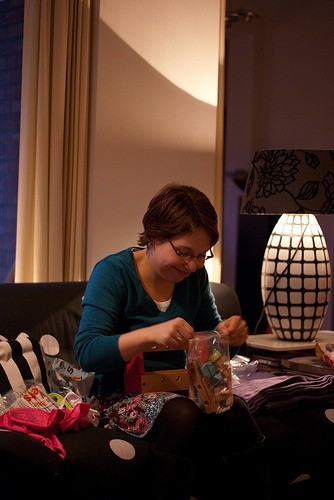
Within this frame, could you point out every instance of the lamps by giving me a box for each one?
[239,148,332,342]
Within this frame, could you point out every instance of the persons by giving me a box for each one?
[73,183,261,500]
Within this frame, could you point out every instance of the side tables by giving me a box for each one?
[245,330,334,375]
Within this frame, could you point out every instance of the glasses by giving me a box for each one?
[168,239,213,260]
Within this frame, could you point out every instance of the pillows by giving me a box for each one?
[0,337,52,408]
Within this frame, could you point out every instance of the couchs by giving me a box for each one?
[0,282,334,475]
